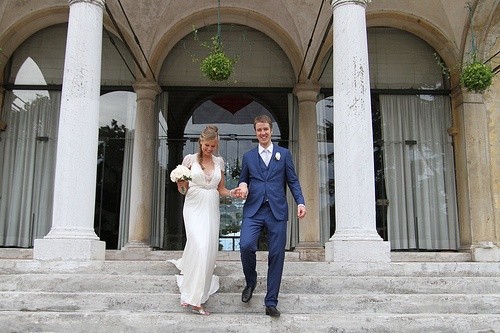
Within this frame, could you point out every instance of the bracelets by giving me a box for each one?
[230,190,233,197]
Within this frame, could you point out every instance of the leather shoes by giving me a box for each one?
[266,305,280,316]
[242,285,256,302]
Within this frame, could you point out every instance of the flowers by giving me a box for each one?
[275,152,281,161]
[170,165,193,193]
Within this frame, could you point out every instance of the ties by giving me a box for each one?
[264,149,270,165]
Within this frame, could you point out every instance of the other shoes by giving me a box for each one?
[180,303,189,307]
[192,306,207,314]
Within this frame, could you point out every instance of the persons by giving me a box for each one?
[236,115,307,317]
[168,126,248,315]
